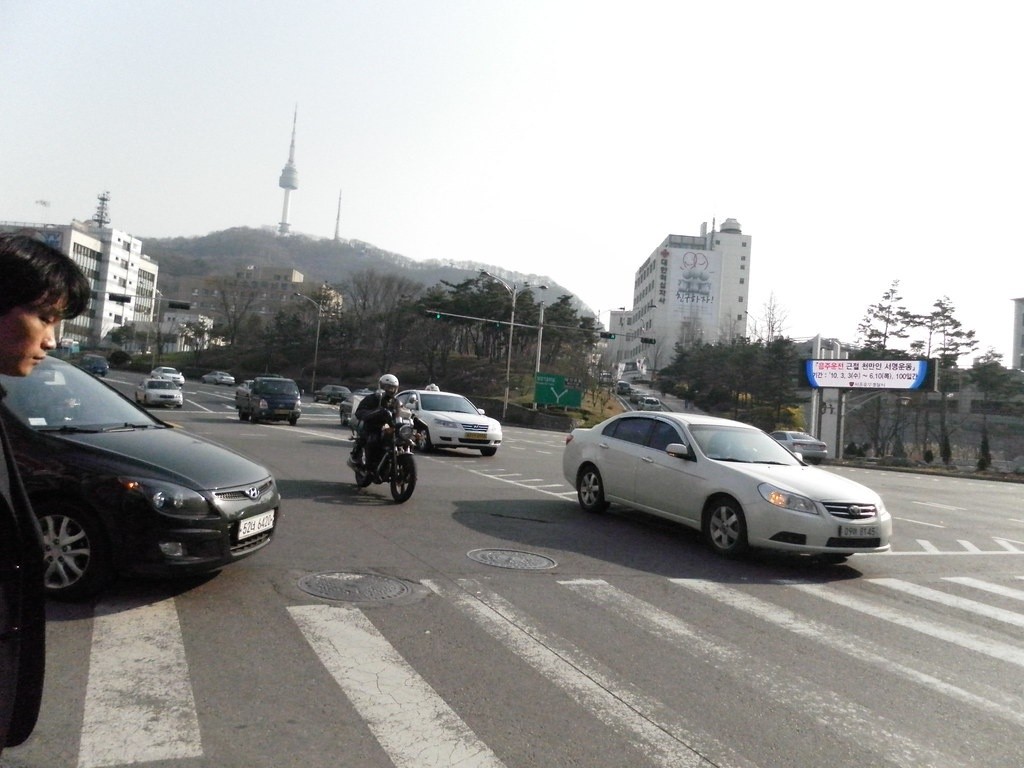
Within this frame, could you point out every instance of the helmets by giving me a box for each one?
[379,374,400,398]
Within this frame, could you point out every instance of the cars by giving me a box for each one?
[73,355,110,376]
[135,366,186,407]
[0,354,281,600]
[393,385,503,457]
[637,397,661,411]
[768,431,829,464]
[236,380,276,399]
[562,410,893,565]
[201,370,234,386]
[599,369,649,402]
[313,385,383,426]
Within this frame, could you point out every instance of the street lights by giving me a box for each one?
[745,311,757,342]
[587,305,657,400]
[294,283,336,394]
[891,395,912,457]
[481,271,548,420]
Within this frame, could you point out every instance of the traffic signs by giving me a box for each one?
[532,371,583,408]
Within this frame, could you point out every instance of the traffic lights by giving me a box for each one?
[641,337,656,344]
[600,332,615,339]
[426,312,441,319]
[485,322,500,328]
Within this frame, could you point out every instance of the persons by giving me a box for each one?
[1,234,88,748]
[355,374,408,475]
[426,377,440,392]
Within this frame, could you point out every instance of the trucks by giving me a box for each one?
[233,377,302,426]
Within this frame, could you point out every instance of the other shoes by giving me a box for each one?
[350,446,363,464]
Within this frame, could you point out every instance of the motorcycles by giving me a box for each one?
[347,395,418,502]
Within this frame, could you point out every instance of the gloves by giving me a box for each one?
[375,406,393,423]
